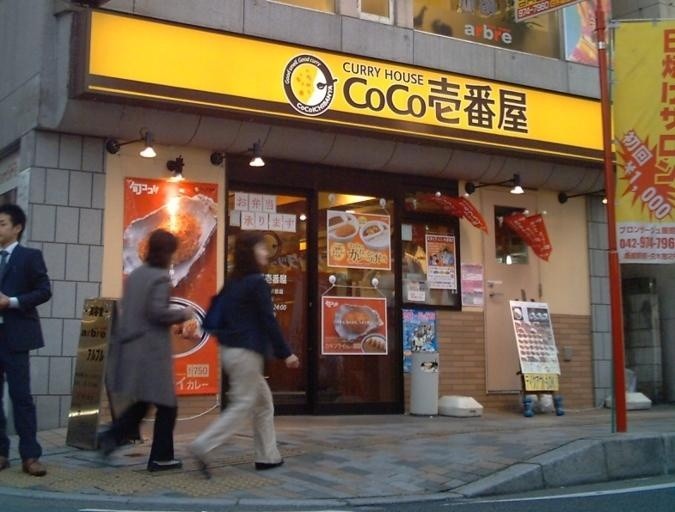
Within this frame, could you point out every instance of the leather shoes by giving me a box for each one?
[148,459,181,471]
[255,459,283,470]
[23,458,46,476]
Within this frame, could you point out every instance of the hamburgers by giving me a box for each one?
[341,311,371,333]
[138,214,200,266]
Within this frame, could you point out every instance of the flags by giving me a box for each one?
[612,16,675,265]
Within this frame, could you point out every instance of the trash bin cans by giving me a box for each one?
[409,352,440,416]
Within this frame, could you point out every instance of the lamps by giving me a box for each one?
[167,156,186,183]
[462,172,522,199]
[555,187,609,209]
[206,138,265,171]
[103,126,158,160]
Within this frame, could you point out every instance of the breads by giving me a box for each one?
[364,337,386,353]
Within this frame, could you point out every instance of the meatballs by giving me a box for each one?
[172,325,182,333]
[183,320,197,337]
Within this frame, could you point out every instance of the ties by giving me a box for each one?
[0,251,8,273]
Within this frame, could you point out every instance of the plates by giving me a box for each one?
[329,213,390,250]
[166,296,210,358]
[359,334,386,353]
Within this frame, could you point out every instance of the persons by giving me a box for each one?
[95,225,196,474]
[188,229,302,482]
[1,204,56,479]
